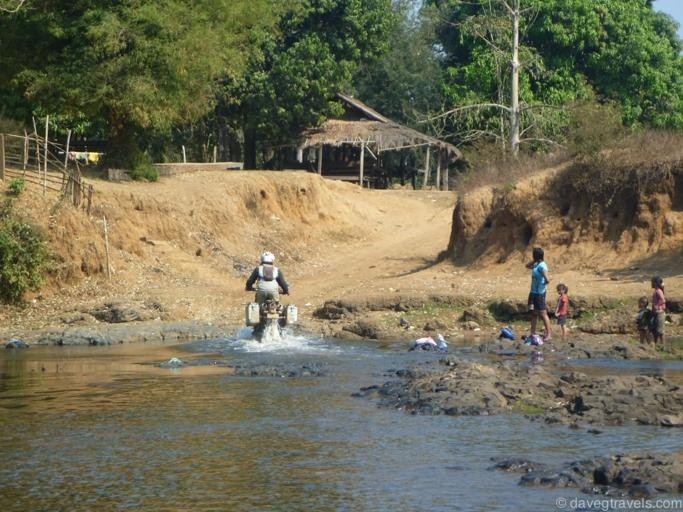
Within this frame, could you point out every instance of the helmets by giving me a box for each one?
[261,251,275,263]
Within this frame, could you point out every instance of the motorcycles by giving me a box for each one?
[245,288,298,343]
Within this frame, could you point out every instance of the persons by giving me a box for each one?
[651,276,666,345]
[245,252,289,336]
[638,296,650,345]
[525,248,552,341]
[555,284,568,340]
